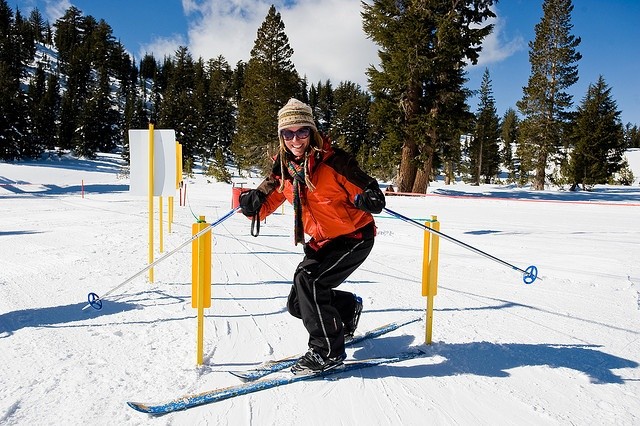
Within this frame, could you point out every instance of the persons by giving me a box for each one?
[239,96,386,378]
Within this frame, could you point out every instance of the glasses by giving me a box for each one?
[280,126,312,141]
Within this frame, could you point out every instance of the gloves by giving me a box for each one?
[240,190,261,217]
[362,185,385,214]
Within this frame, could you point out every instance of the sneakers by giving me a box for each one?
[290,348,346,376]
[342,294,362,341]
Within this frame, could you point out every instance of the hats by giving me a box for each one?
[277,98,316,193]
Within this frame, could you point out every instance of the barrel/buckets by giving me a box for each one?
[232,187,251,213]
